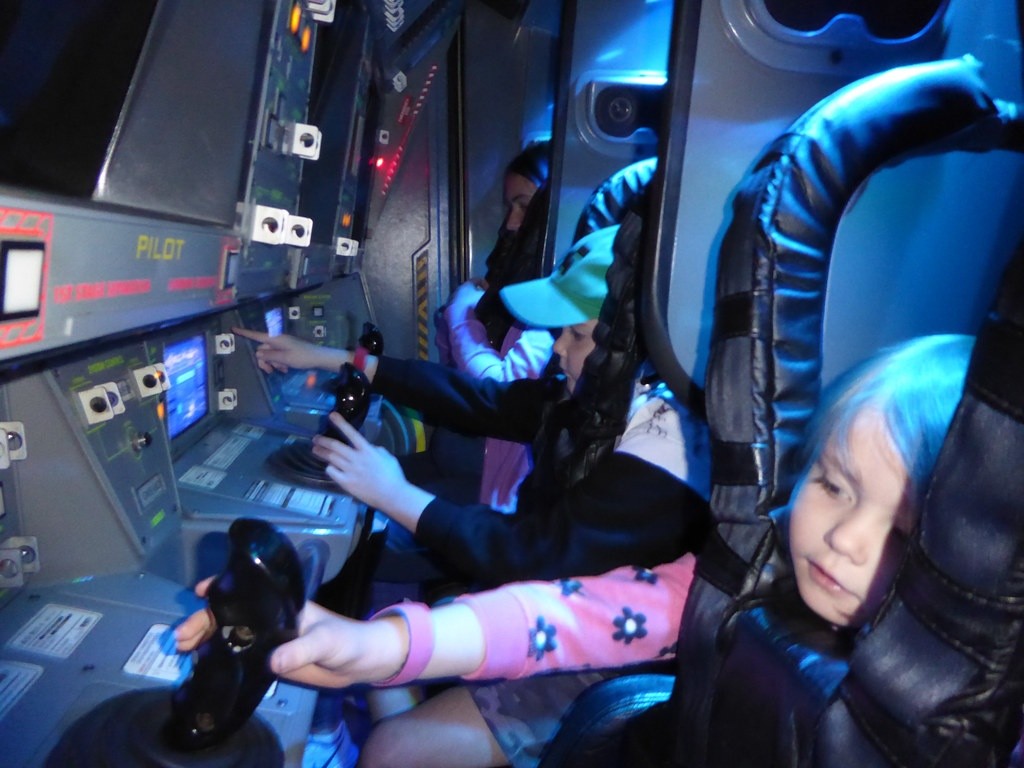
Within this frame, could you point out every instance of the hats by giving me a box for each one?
[497,223,621,330]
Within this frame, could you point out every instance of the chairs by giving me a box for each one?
[527,59,1024,766]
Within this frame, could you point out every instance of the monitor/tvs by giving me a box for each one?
[161,325,215,451]
[259,301,287,339]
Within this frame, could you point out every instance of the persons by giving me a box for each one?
[217,225,716,768]
[168,335,978,768]
[349,231,558,587]
[476,138,552,290]
